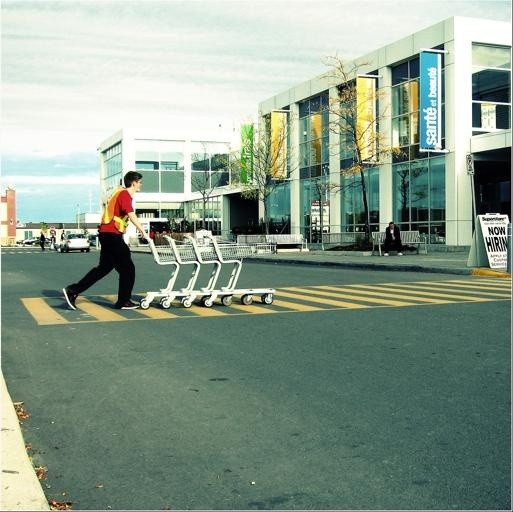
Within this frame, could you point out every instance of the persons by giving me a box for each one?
[61,171,148,311]
[39,232,45,250]
[384,222,402,256]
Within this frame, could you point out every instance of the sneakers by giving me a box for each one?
[382,252,404,257]
[63,284,80,311]
[113,299,140,310]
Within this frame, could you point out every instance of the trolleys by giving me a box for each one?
[140,232,277,310]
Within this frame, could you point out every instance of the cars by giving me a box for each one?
[92,240,96,245]
[60,233,90,252]
[430,230,445,243]
[17,237,51,245]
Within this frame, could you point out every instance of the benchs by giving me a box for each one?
[237,234,309,253]
[322,231,427,257]
